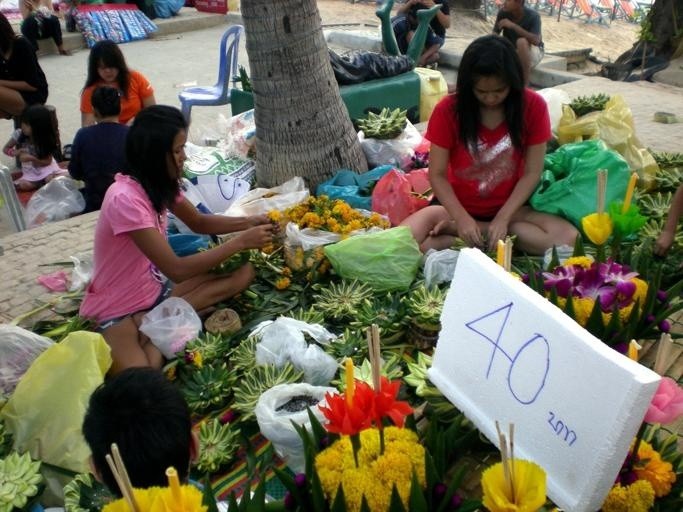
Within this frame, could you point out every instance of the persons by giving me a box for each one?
[80,106,280,374]
[18,0,69,55]
[391,0,450,69]
[0,13,48,119]
[399,34,582,259]
[652,182,683,258]
[83,365,202,501]
[2,105,60,191]
[493,0,545,80]
[79,40,156,127]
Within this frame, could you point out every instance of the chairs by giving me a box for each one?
[178,26,243,139]
